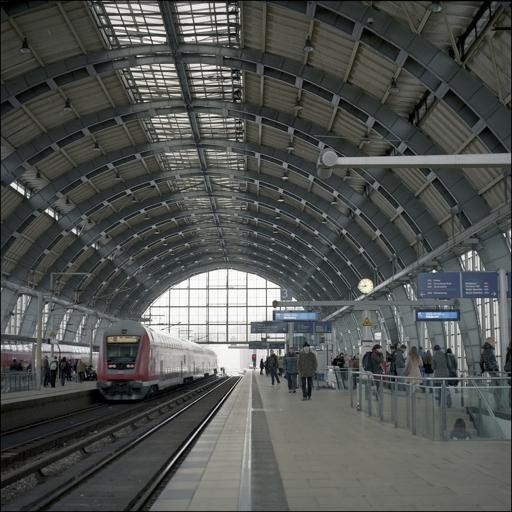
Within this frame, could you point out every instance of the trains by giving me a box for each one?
[95,319,220,401]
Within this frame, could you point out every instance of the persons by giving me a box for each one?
[478,336,505,410]
[10,355,97,388]
[331,341,458,408]
[449,417,471,441]
[259,342,323,400]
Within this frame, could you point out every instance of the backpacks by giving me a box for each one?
[362,352,372,371]
[60,362,66,370]
[268,355,276,370]
[50,362,57,370]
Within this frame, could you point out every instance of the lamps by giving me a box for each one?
[391,76,400,93]
[92,139,99,151]
[287,141,294,150]
[19,37,31,54]
[357,277,375,296]
[344,168,352,179]
[302,35,316,53]
[104,233,110,240]
[115,172,120,180]
[87,218,93,225]
[361,129,372,145]
[293,96,304,110]
[143,212,150,220]
[331,197,338,206]
[131,195,136,203]
[281,170,289,181]
[63,96,73,113]
[429,1,442,14]
[35,171,42,180]
[65,195,72,206]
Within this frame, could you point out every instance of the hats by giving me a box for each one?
[485,337,496,349]
[303,342,310,347]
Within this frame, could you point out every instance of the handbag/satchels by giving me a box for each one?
[403,367,409,376]
[391,360,395,370]
[423,364,433,373]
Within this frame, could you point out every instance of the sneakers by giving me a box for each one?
[302,396,312,400]
[288,390,296,393]
